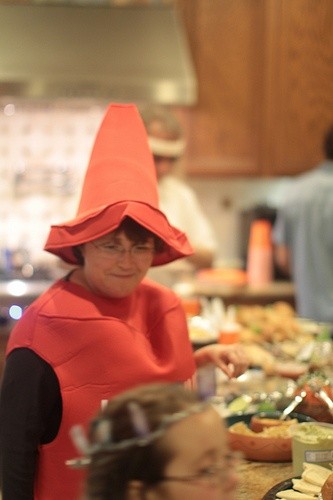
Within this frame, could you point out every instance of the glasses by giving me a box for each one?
[91,240,159,261]
[162,451,239,488]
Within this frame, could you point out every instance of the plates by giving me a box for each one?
[261,476,305,500]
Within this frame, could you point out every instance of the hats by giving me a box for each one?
[43,102,194,266]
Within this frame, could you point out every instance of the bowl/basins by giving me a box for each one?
[223,411,318,462]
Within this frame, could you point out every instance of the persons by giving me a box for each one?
[271,121,333,324]
[144,110,218,269]
[88,383,239,500]
[0,193,197,500]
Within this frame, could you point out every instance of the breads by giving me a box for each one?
[276,463,333,500]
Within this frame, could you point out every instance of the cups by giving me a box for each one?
[291,421,333,475]
